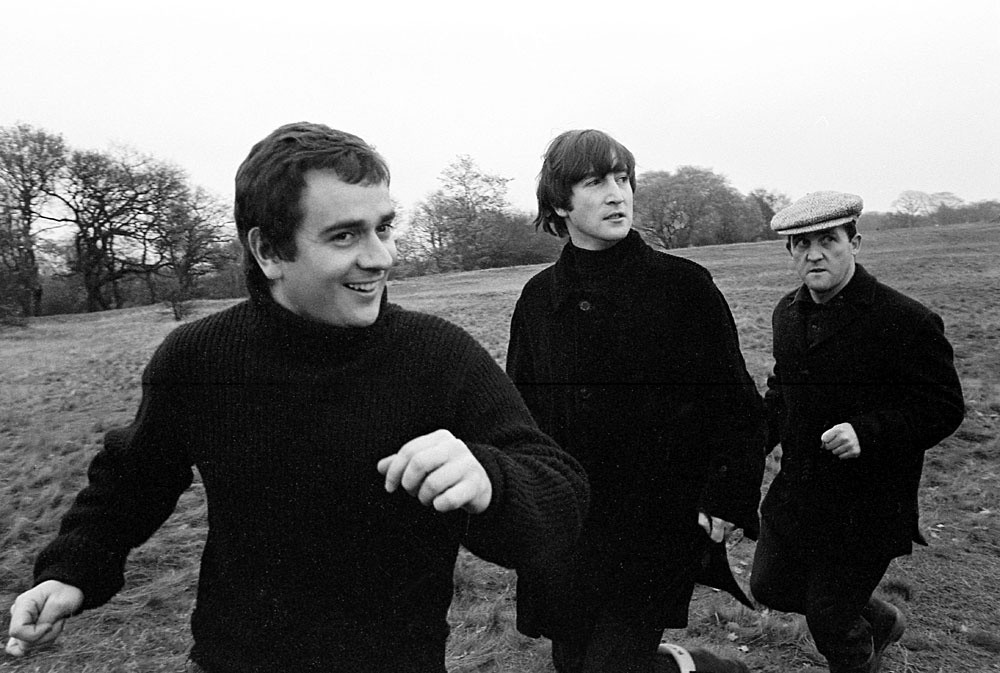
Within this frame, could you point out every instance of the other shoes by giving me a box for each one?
[870,600,907,668]
[721,657,749,673]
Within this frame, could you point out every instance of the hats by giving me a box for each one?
[771,191,864,238]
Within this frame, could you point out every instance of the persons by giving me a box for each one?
[505,131,767,673]
[7,120,592,673]
[750,190,962,673]
